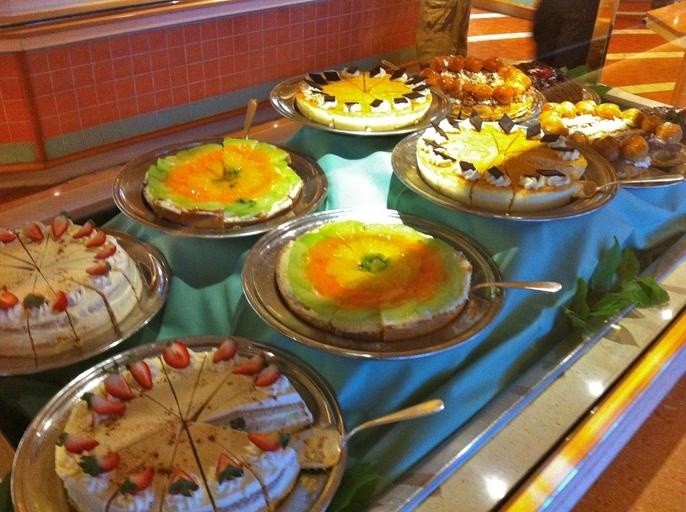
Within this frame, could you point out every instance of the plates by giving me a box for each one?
[389,117,618,224]
[269,66,439,134]
[420,49,543,126]
[2,212,176,377]
[112,133,331,241]
[244,203,509,364]
[8,336,348,511]
[620,106,686,194]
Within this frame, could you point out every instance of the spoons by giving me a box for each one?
[473,280,567,295]
[589,172,686,199]
[285,393,453,481]
[238,100,267,139]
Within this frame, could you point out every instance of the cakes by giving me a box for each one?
[54,337,312,512]
[539,98,686,175]
[275,219,472,341]
[142,136,303,230]
[415,108,587,214]
[295,63,433,133]
[516,59,584,104]
[0,214,142,357]
[419,54,537,123]
